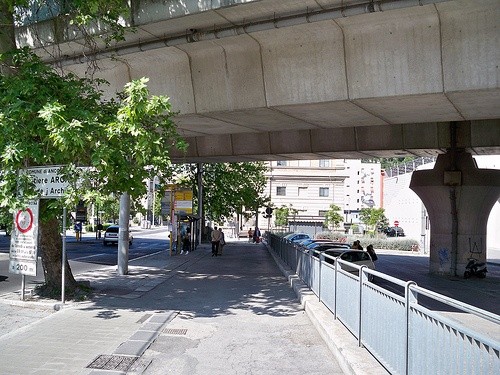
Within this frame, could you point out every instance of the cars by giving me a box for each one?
[282,233,350,261]
[103,226,133,246]
[315,248,376,282]
[388,226,403,236]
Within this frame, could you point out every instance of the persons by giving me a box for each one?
[247,227,253,242]
[97,223,103,239]
[210,227,222,257]
[351,241,358,260]
[252,227,261,244]
[364,245,380,284]
[356,239,364,260]
[74,219,83,242]
[217,228,224,256]
[180,227,192,256]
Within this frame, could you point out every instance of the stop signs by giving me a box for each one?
[394,221,399,226]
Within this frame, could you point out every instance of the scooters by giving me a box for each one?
[463,257,488,279]
